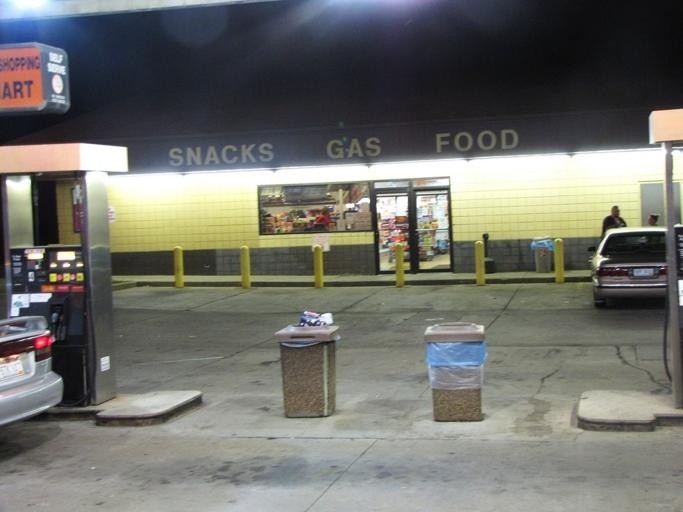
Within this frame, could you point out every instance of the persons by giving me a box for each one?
[599,206,627,242]
[648,214,659,227]
[315,207,330,226]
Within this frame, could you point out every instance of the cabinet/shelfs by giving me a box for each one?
[381,228,439,262]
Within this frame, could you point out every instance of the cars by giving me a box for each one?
[0,314,64,427]
[588,225,668,308]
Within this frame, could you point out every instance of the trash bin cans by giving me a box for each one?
[275,324,342,418]
[531,239,552,273]
[424,320,485,422]
